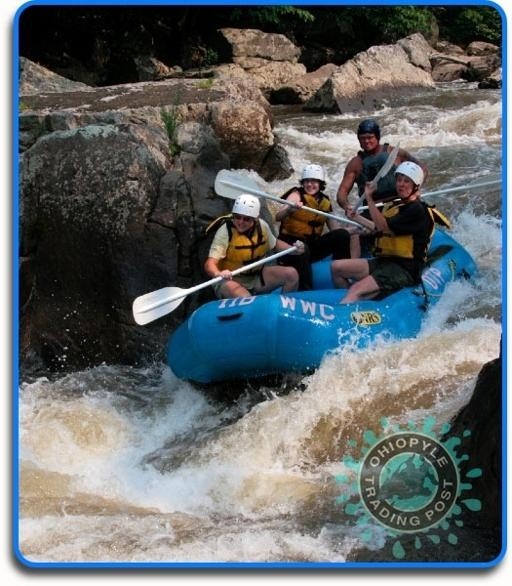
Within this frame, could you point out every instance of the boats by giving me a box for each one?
[168,224,478,390]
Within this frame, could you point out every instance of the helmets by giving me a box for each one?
[229,192,263,219]
[355,118,381,140]
[299,163,326,183]
[392,160,425,188]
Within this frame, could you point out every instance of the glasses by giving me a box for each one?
[233,213,251,222]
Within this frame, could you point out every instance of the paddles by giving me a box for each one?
[214,169,364,229]
[132,246,298,326]
[352,142,400,211]
[356,173,503,210]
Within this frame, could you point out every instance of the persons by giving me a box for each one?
[335,118,429,258]
[329,159,435,305]
[200,192,306,301]
[274,163,351,292]
